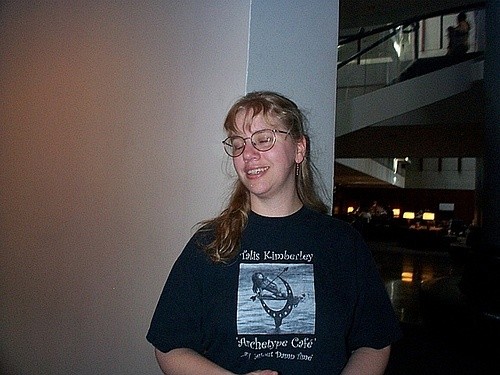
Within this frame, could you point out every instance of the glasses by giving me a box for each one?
[222,129,290,157]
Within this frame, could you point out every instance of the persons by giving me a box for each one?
[446,10,471,57]
[145,92,397,372]
[251,272,288,301]
